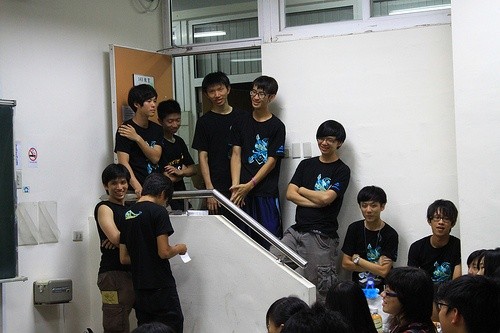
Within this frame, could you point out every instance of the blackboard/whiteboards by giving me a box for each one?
[0,98,28,286]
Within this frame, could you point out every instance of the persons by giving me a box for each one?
[408,200,461,321]
[341,186,399,333]
[270,120,351,302]
[227,75,286,248]
[328,280,377,333]
[466,248,500,277]
[94,162,134,333]
[192,72,246,227]
[157,99,197,212]
[119,173,187,333]
[283,302,351,333]
[437,276,500,333]
[266,296,308,333]
[381,267,439,333]
[114,84,172,211]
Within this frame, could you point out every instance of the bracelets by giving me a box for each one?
[352,256,360,264]
[252,178,256,186]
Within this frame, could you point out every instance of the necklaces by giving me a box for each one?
[364,220,381,252]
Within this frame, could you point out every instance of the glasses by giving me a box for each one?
[317,136,337,145]
[437,301,449,311]
[250,91,270,99]
[383,284,398,298]
[430,215,452,223]
